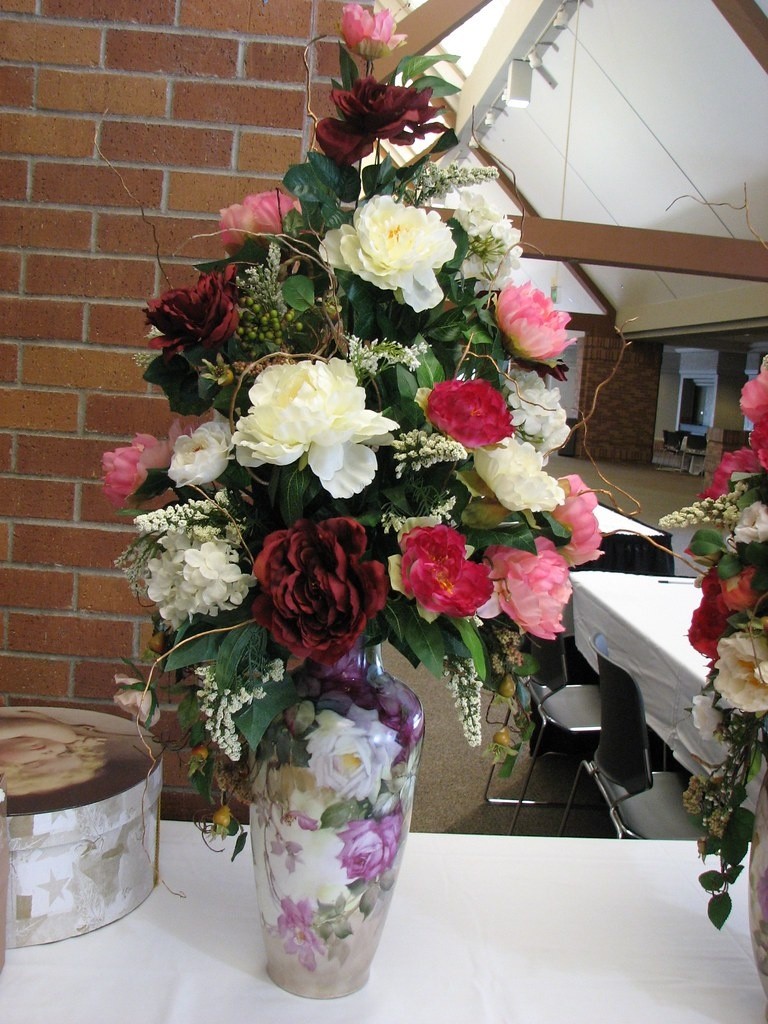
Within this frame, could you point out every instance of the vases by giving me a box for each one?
[749,769,768,996]
[249,633,424,998]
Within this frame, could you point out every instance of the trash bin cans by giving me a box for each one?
[559,418,576,456]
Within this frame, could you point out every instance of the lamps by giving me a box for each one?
[469,0,573,148]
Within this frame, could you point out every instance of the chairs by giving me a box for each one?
[485,614,709,840]
[655,430,708,474]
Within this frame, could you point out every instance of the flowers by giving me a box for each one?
[96,4,606,855]
[657,358,768,933]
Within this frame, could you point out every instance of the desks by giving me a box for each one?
[577,500,675,577]
[1,820,768,1024]
[571,568,768,809]
